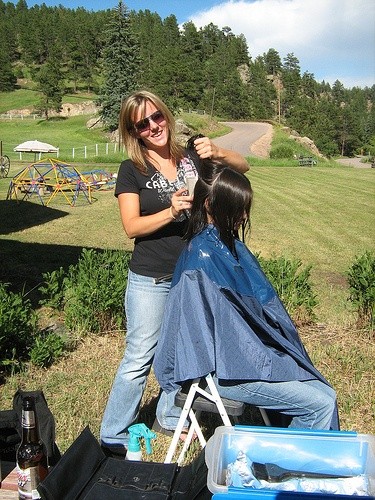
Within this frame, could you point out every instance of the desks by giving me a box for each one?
[0,464,50,500]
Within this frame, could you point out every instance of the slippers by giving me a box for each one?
[100,443,126,460]
[152,416,200,446]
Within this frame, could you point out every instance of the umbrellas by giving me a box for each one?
[13,140,59,163]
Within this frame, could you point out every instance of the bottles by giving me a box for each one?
[16,397,48,500]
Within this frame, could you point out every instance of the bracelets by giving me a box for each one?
[170,206,176,219]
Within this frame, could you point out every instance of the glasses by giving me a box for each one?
[134,109,165,135]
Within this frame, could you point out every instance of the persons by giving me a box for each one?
[100,91,250,461]
[152,133,341,431]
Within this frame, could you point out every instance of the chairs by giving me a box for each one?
[162,373,272,466]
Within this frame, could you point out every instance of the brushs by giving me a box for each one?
[251,462,357,482]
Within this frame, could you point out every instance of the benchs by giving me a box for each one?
[14,173,118,194]
[299,156,316,167]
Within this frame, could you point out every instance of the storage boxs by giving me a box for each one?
[204,424,375,500]
